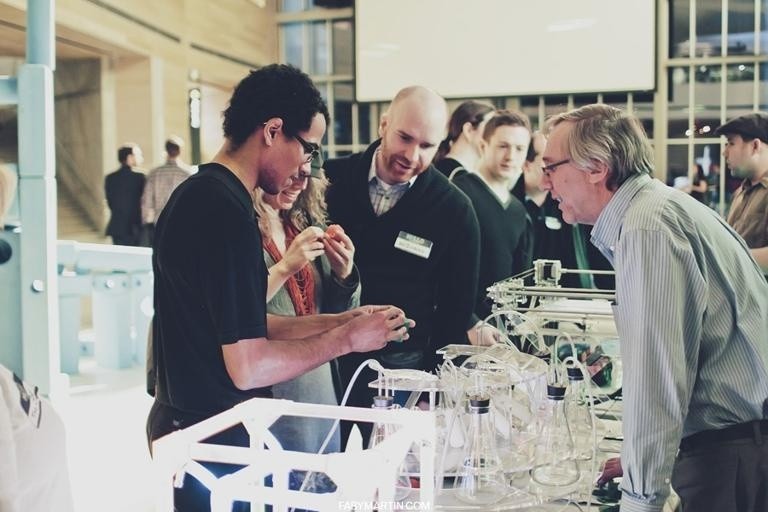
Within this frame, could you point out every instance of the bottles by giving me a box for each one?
[567,363,595,459]
[367,395,413,501]
[451,391,507,505]
[530,383,580,487]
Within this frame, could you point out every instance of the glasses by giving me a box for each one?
[541,160,570,177]
[263,122,318,162]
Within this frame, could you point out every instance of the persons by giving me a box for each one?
[1,143,69,511]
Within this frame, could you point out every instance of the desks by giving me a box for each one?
[393,401,686,512]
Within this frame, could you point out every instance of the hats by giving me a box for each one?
[716,113,768,141]
[300,143,326,177]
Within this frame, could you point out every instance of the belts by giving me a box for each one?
[679,420,767,453]
